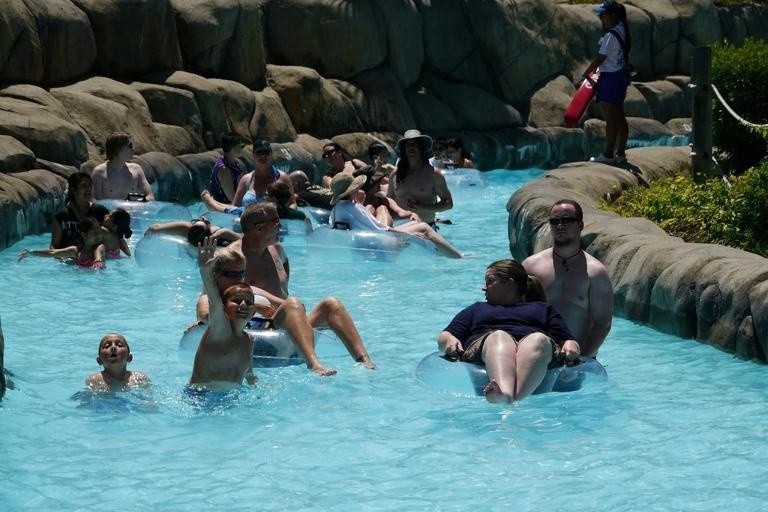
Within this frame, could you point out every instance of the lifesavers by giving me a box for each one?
[180,319,314,368]
[306,205,331,226]
[415,352,608,395]
[91,198,192,232]
[308,225,439,261]
[198,202,313,252]
[441,168,486,193]
[134,233,228,274]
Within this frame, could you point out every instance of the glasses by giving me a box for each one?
[253,215,279,226]
[548,217,577,225]
[322,149,335,158]
[221,270,245,279]
[190,219,210,227]
[125,142,132,148]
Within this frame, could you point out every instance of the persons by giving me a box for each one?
[197,201,376,378]
[144,216,243,248]
[435,258,581,405]
[86,332,150,393]
[319,129,464,259]
[521,199,614,359]
[188,236,259,387]
[200,132,309,221]
[575,0,631,167]
[18,171,133,273]
[90,131,158,201]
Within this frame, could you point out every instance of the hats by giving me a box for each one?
[329,171,367,206]
[352,164,387,192]
[395,129,433,153]
[253,139,271,153]
[592,0,612,12]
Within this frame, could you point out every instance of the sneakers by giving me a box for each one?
[615,154,626,164]
[589,152,615,165]
